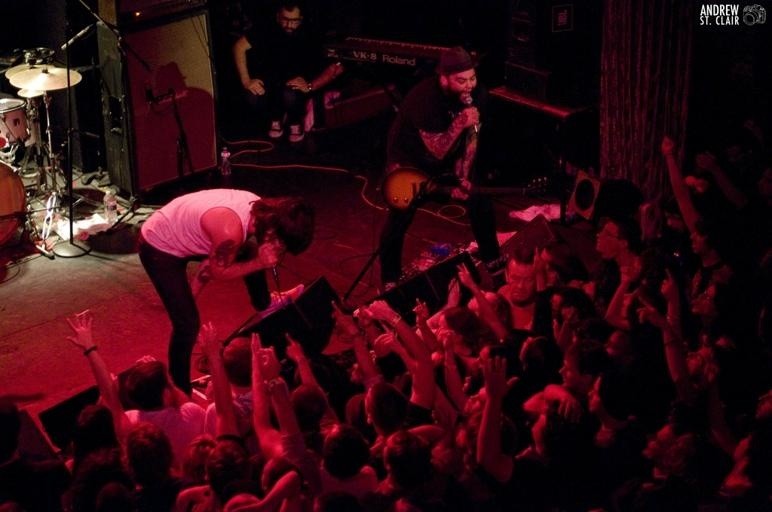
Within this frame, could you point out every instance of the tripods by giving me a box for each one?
[27,92,104,218]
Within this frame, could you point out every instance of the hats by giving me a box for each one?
[432,45,479,76]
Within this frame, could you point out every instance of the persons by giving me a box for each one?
[233,1,354,143]
[2,117,772,511]
[376,44,508,300]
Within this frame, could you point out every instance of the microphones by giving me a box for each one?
[459,92,479,136]
[262,243,280,288]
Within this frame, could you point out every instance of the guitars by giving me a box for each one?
[382,162,553,211]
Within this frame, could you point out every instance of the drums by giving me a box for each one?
[0,97,30,148]
[0,160,26,248]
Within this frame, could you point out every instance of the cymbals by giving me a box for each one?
[16,88,49,98]
[10,67,81,88]
[5,64,55,81]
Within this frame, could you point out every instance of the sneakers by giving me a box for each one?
[258,284,305,320]
[383,280,399,293]
[268,112,305,144]
[486,255,510,274]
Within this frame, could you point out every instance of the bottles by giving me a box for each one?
[103,190,117,225]
[220,147,231,176]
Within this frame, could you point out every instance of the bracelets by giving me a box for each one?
[307,82,312,91]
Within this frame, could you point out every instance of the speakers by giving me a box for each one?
[222,275,339,362]
[477,213,590,293]
[38,366,131,455]
[363,250,481,326]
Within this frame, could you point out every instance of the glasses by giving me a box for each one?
[280,15,300,25]
